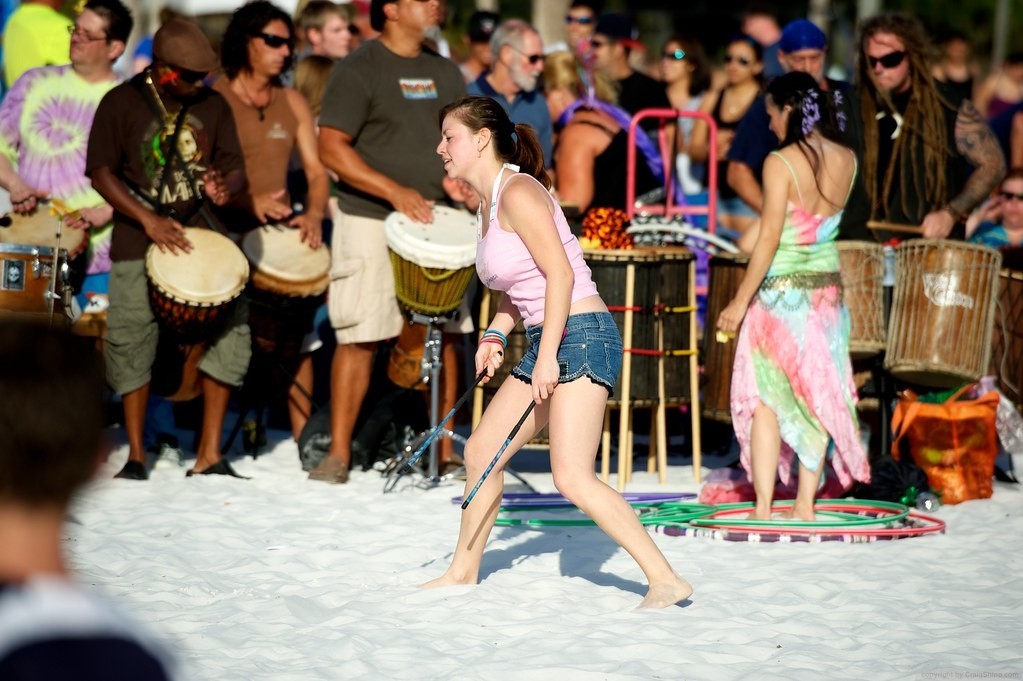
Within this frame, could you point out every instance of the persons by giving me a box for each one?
[460,10,502,83]
[420,96,693,612]
[1,0,468,519]
[0,323,184,681]
[543,3,1023,524]
[467,18,557,192]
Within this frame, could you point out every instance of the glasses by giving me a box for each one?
[868,49,907,69]
[998,191,1023,202]
[66,24,107,44]
[659,49,689,61]
[589,40,610,49]
[563,15,593,24]
[527,54,548,64]
[258,32,295,50]
[723,54,749,66]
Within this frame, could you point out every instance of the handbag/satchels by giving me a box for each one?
[887,376,1023,506]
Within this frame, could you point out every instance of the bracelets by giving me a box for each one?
[477,330,510,349]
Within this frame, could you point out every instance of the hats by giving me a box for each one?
[152,9,222,71]
[465,9,503,44]
[779,20,825,54]
[597,15,652,54]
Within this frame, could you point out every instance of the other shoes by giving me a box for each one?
[113,460,151,487]
[185,459,249,480]
[299,435,329,472]
[420,453,468,479]
[308,456,349,483]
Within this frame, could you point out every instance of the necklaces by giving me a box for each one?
[236,74,273,121]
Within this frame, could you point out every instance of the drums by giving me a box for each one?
[240,220,334,354]
[883,236,1003,390]
[2,200,92,262]
[582,246,699,408]
[143,226,252,404]
[702,251,751,424]
[384,203,479,392]
[482,200,583,448]
[831,239,888,360]
[0,242,74,323]
